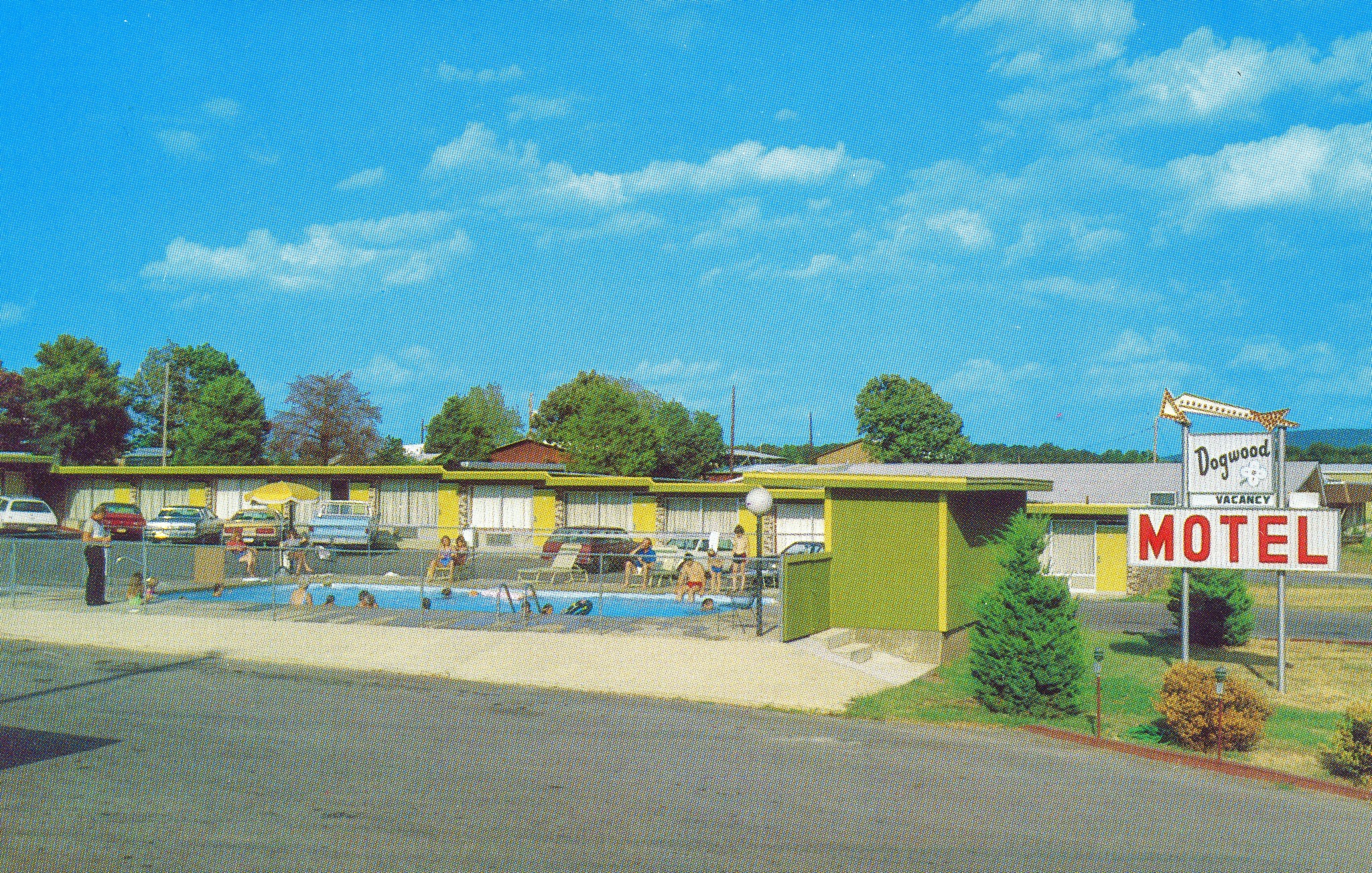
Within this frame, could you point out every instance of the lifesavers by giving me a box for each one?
[561,599,592,616]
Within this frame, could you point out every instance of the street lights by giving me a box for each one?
[743,487,773,638]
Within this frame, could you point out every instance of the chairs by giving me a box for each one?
[518,543,589,587]
[626,545,679,588]
[431,546,469,582]
[722,561,777,591]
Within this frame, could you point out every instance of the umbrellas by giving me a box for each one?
[245,479,316,505]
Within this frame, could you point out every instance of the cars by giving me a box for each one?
[222,508,292,547]
[743,541,824,588]
[540,526,644,573]
[308,499,384,548]
[91,502,149,541]
[143,505,224,544]
[0,494,60,535]
[649,535,739,576]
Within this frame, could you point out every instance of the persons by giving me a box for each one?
[285,529,312,574]
[707,550,723,594]
[623,538,656,590]
[229,531,258,578]
[82,505,113,606]
[732,525,750,591]
[702,598,714,609]
[430,534,467,577]
[676,554,705,603]
